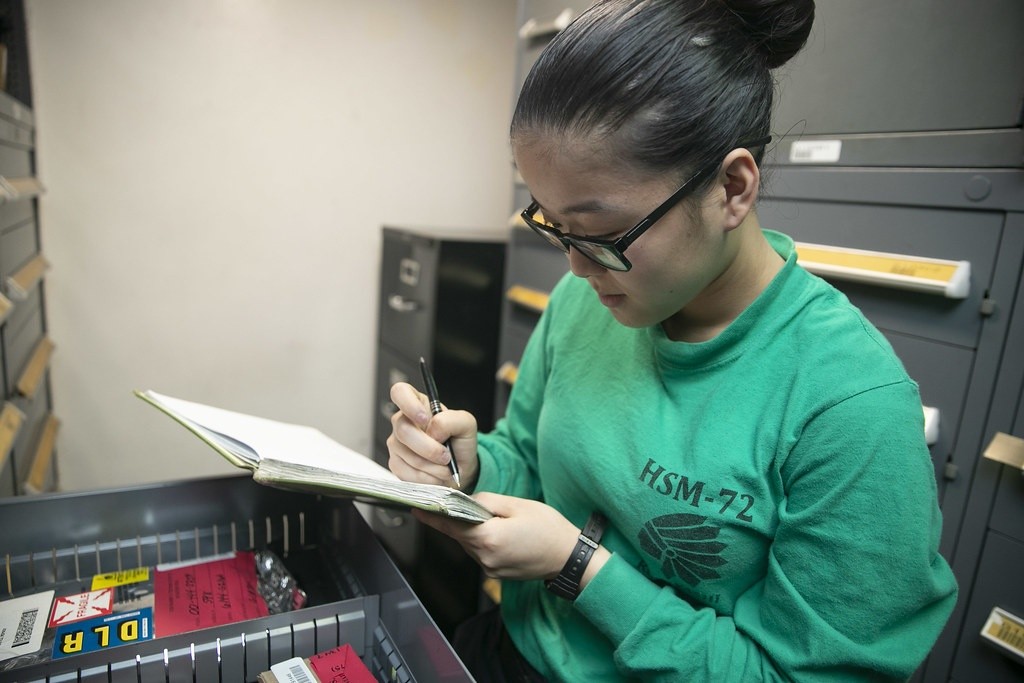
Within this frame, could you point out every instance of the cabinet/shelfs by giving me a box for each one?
[373,221,508,470]
[472,0,1024,683]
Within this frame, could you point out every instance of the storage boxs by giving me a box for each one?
[0,0,62,498]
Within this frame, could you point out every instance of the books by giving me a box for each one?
[136,388,491,525]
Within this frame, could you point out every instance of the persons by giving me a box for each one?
[387,0,960,683]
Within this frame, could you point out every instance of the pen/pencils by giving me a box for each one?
[419,355,461,487]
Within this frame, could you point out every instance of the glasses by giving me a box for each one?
[521,134,773,272]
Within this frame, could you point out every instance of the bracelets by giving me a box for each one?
[543,511,608,603]
